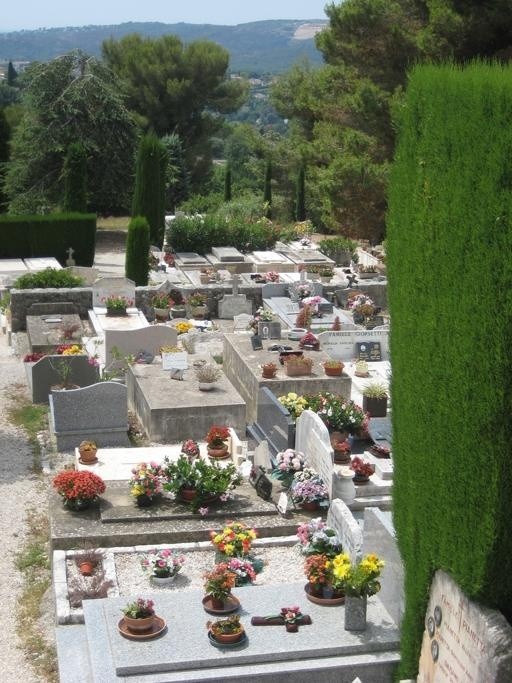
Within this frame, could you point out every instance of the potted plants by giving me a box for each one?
[74,539,104,575]
[206,613,247,647]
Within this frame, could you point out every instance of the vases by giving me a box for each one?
[210,586,228,610]
[150,575,177,583]
[123,612,155,631]
[343,590,367,630]
[309,574,334,598]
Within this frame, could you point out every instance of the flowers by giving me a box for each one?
[225,553,257,587]
[326,550,385,597]
[199,561,237,600]
[23,232,390,510]
[304,549,339,598]
[208,518,259,561]
[138,545,186,576]
[122,595,158,618]
[294,516,342,557]
